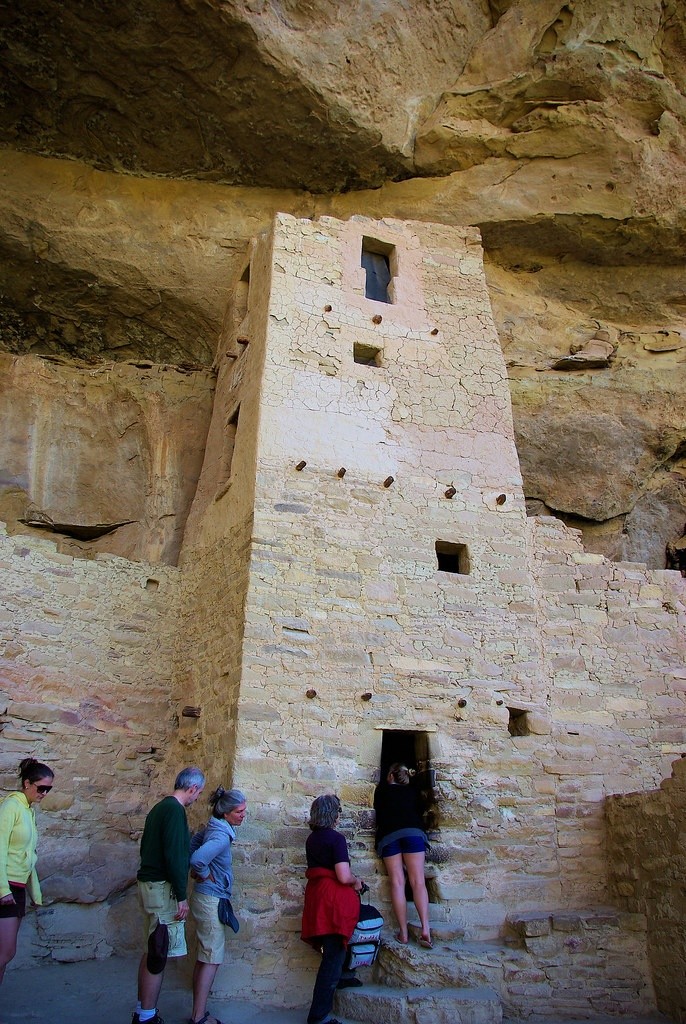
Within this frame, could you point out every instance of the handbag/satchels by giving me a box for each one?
[341,883,385,971]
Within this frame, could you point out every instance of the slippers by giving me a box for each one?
[418,936,434,949]
[394,934,407,944]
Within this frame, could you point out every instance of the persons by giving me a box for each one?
[300,794,369,1024]
[0,757,55,986]
[130,766,204,1024]
[190,785,246,1024]
[371,764,436,950]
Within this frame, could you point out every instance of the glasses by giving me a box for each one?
[32,782,53,794]
[337,807,342,814]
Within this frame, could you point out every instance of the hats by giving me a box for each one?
[147,924,170,974]
[218,898,240,933]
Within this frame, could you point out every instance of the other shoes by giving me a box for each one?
[336,977,363,989]
[131,1008,166,1024]
[189,1011,222,1024]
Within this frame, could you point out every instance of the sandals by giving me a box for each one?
[324,1017,342,1024]
